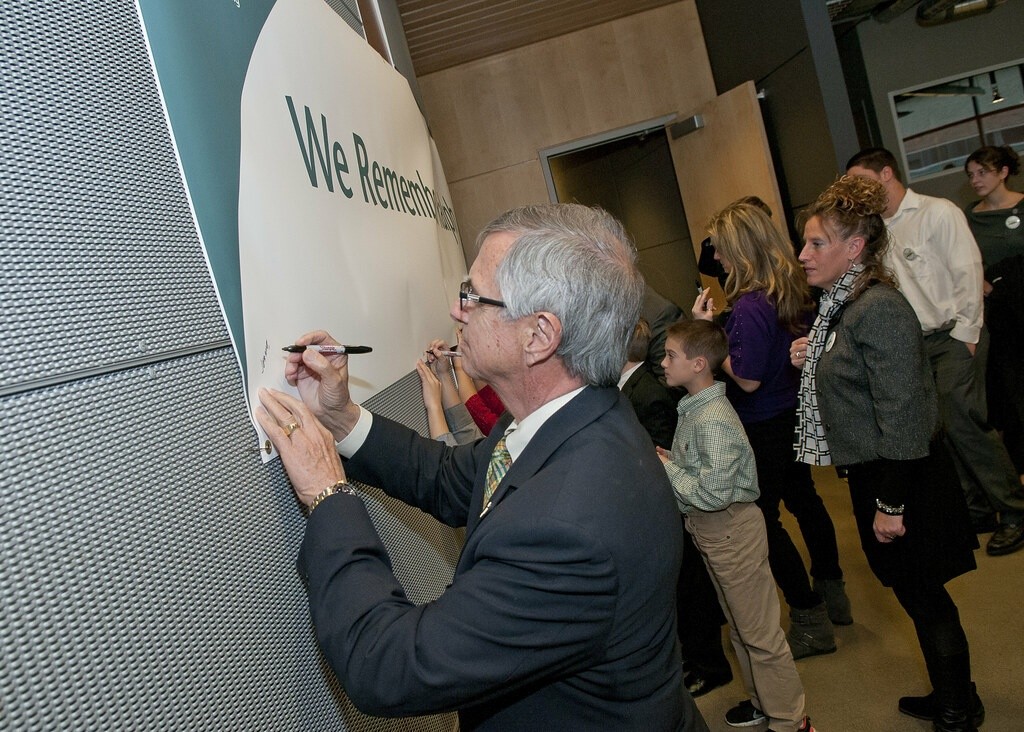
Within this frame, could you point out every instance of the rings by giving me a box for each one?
[284,421,300,436]
[890,536,894,540]
[796,351,801,359]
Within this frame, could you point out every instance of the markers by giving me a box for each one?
[426,350,462,356]
[281,345,373,354]
[694,278,707,309]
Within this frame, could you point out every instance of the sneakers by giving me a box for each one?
[724,698,770,727]
[765,715,816,732]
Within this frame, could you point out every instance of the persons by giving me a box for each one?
[616,317,735,694]
[845,148,1024,556]
[790,174,986,731]
[964,145,1024,488]
[416,327,508,446]
[658,320,814,732]
[255,204,709,732]
[692,196,854,659]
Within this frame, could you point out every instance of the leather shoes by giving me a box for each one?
[987,522,1024,555]
[967,513,999,533]
[678,645,733,697]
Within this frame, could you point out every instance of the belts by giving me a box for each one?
[924,319,955,337]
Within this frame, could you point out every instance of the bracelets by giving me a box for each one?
[876,498,904,514]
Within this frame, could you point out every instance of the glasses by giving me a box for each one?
[459,281,507,311]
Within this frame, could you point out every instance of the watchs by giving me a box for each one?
[308,480,357,518]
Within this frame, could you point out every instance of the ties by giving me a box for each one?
[483,432,512,514]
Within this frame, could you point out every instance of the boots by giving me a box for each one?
[811,576,854,625]
[784,602,837,660]
[899,647,985,732]
